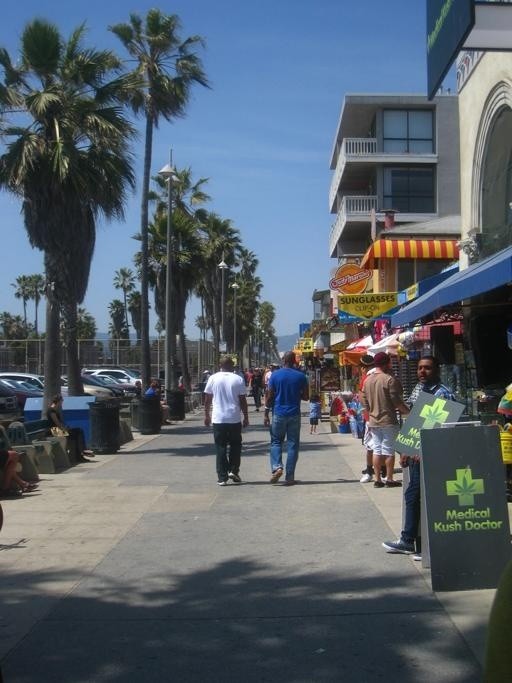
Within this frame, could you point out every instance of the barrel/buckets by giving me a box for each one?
[166,388,186,421]
[138,396,161,435]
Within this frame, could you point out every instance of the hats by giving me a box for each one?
[360,355,375,366]
[374,352,390,366]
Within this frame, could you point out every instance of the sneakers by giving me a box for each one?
[360,473,402,487]
[270,468,283,484]
[228,470,241,481]
[285,479,297,485]
[217,481,226,485]
[382,540,415,555]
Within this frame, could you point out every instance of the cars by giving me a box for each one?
[0,366,166,414]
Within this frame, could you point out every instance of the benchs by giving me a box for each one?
[6,418,82,473]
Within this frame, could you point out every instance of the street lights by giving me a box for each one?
[158,147,183,391]
[217,249,229,354]
[253,313,280,367]
[231,273,240,353]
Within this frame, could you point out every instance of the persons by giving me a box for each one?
[308,389,323,435]
[1,424,40,493]
[135,360,313,425]
[201,354,251,489]
[263,349,310,485]
[358,352,463,562]
[46,394,95,462]
[0,450,20,493]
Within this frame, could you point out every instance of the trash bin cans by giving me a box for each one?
[164,389,184,420]
